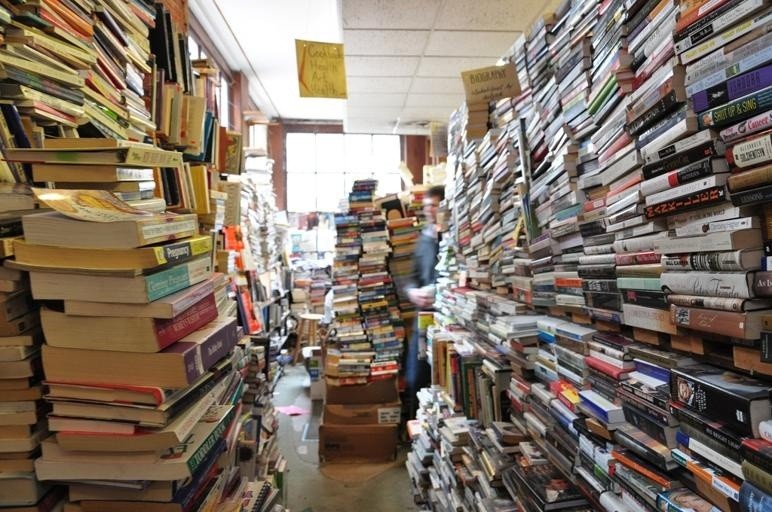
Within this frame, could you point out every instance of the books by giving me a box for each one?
[405,0,771,511]
[289,178,422,385]
[2,1,289,511]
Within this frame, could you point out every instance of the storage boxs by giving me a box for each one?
[315,373,403,464]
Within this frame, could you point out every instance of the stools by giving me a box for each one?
[292,313,327,365]
